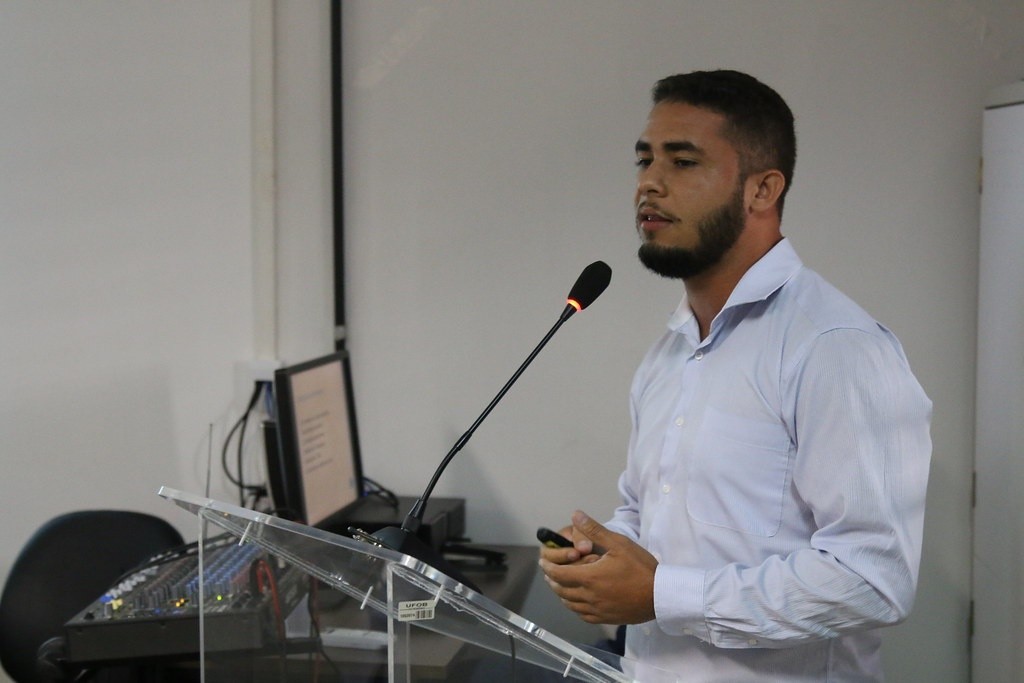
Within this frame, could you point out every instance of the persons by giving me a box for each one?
[537,69,935,683]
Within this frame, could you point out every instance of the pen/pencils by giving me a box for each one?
[536,527,574,548]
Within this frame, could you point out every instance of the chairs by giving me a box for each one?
[0,508,190,683]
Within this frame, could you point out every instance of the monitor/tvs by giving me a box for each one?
[261,349,364,542]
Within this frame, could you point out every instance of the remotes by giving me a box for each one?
[536,527,575,550]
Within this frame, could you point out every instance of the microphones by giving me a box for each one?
[370,261,614,596]
